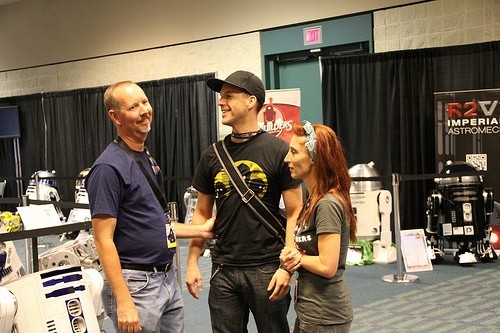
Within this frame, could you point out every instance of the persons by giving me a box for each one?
[185,70,304,333]
[85,81,185,333]
[279,119,358,333]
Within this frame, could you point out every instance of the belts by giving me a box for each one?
[121,262,173,273]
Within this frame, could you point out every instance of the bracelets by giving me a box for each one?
[279,264,294,275]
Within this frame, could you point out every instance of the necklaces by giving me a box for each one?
[304,183,318,211]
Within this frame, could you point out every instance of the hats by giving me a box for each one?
[207,70,265,104]
